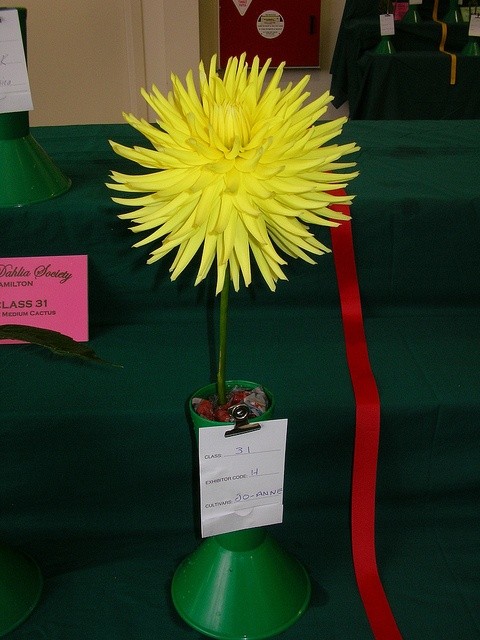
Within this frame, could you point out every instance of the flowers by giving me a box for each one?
[106,51,361,403]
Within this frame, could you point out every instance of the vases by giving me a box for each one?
[170,380,316,640]
